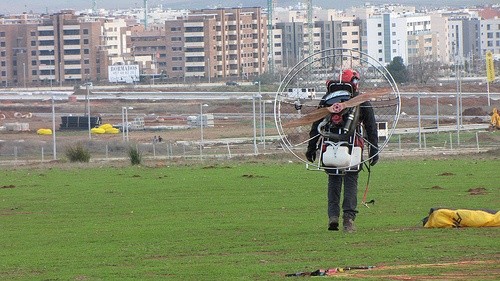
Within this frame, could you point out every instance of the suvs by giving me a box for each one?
[225,81,240,86]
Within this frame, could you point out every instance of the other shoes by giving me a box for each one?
[328,216,339,231]
[344,219,357,232]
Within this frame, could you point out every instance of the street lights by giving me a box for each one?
[200,103,208,162]
[208,58,210,82]
[85,82,92,137]
[296,77,303,120]
[252,81,272,154]
[455,55,471,144]
[121,106,133,141]
[22,62,26,90]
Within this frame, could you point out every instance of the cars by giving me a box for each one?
[37,129,53,135]
[119,126,132,132]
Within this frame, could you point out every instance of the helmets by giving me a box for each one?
[337,70,361,94]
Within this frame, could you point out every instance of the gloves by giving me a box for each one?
[305,147,316,163]
[369,151,379,166]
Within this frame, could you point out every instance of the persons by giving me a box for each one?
[305,69,379,233]
